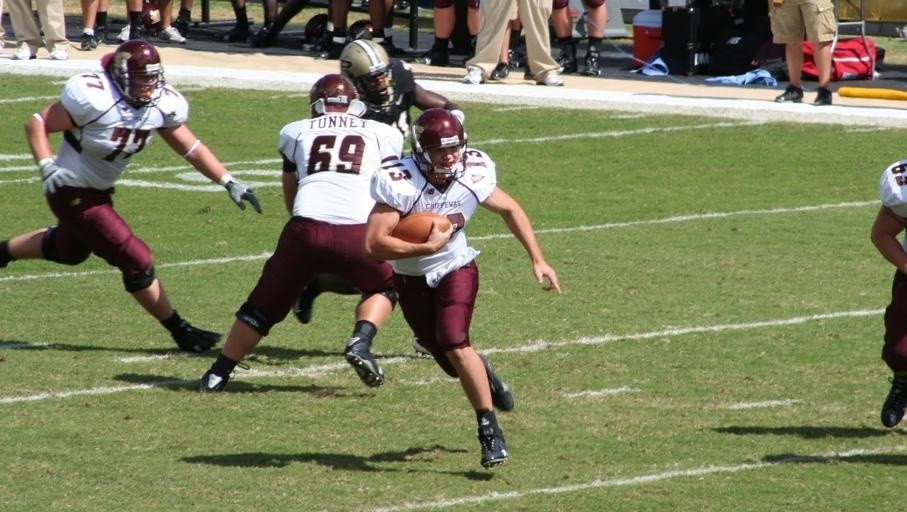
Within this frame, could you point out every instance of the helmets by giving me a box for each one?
[409,108,468,180]
[107,39,165,108]
[302,13,398,115]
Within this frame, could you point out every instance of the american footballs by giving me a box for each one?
[392,211,454,243]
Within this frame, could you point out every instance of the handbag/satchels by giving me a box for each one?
[800,36,876,81]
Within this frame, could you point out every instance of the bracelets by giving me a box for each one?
[39,158,54,167]
[450,109,464,125]
[219,174,234,186]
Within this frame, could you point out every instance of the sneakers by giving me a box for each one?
[200,364,230,394]
[81,24,187,51]
[344,334,384,387]
[881,375,906,427]
[413,50,450,67]
[292,292,315,326]
[171,318,221,352]
[479,354,514,411]
[775,85,803,101]
[12,44,69,60]
[814,87,832,105]
[463,52,600,87]
[222,23,276,48]
[478,429,508,468]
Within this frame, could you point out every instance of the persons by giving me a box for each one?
[200,74,404,391]
[1,1,837,105]
[871,158,907,428]
[363,108,561,468]
[0,40,262,353]
[293,40,466,355]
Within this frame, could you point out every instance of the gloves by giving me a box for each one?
[444,102,465,125]
[220,174,263,215]
[39,157,77,194]
